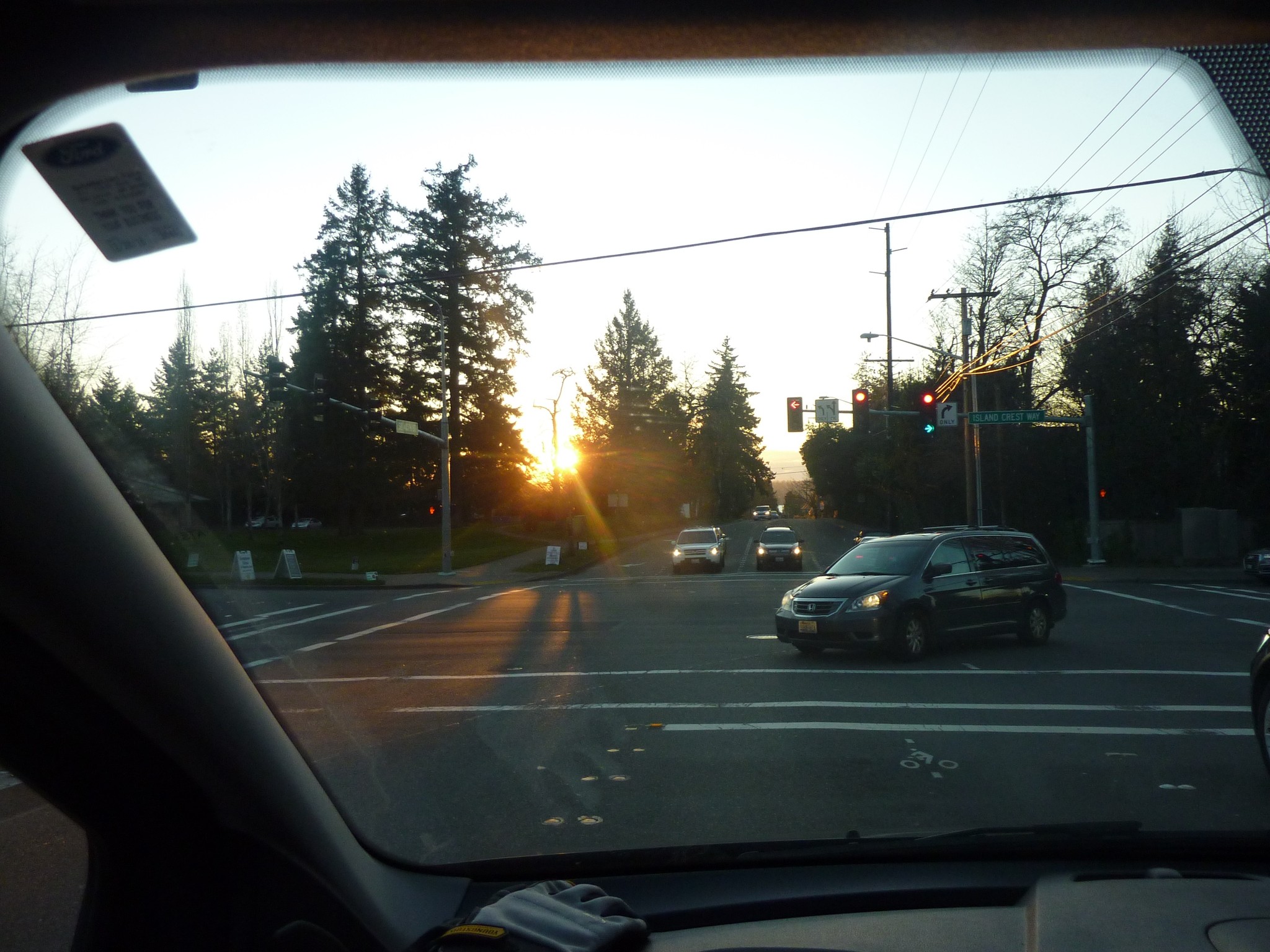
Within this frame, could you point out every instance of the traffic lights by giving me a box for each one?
[428,504,438,516]
[267,355,289,403]
[853,388,870,433]
[1099,484,1110,498]
[787,396,805,433]
[919,392,937,444]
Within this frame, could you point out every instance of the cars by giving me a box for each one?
[246,516,280,529]
[292,517,322,530]
[1247,626,1270,779]
[1242,544,1270,587]
[753,505,772,521]
[770,511,780,520]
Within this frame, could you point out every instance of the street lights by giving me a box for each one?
[373,269,456,577]
[860,331,975,527]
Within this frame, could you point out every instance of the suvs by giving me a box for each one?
[667,525,729,573]
[753,525,805,569]
[774,524,1067,664]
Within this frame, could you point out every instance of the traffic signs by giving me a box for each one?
[814,398,840,423]
[936,402,959,427]
